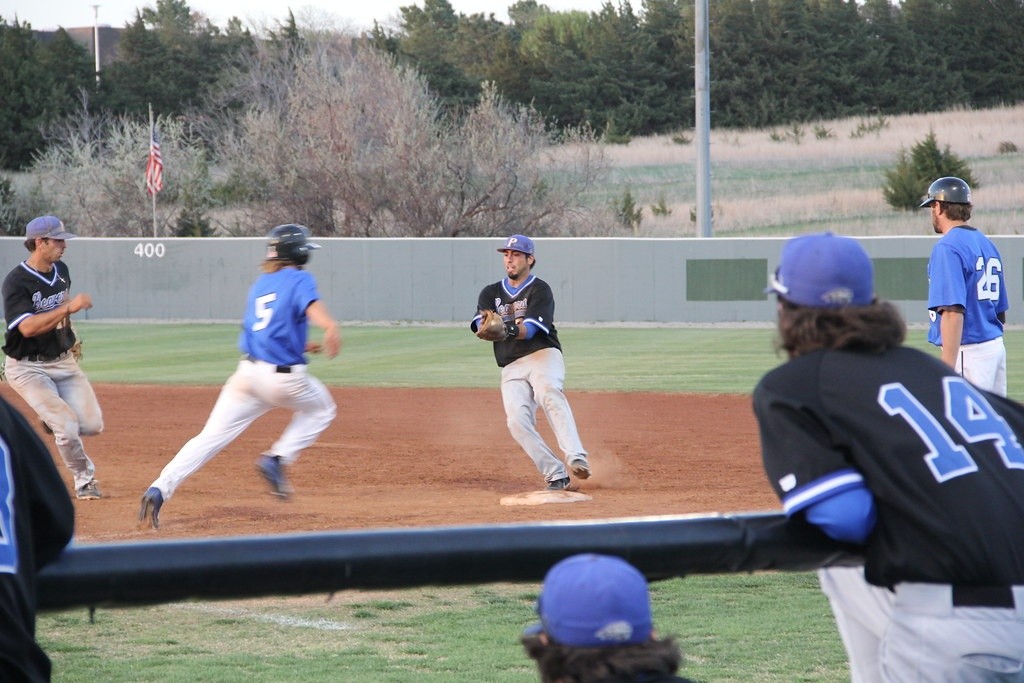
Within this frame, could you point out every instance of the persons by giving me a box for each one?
[752,233,1024,683]
[0,396,74,683]
[471,234,590,490]
[518,553,692,683]
[0,216,103,500]
[919,178,1009,395]
[139,224,341,529]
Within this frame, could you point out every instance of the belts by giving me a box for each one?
[951,583,1016,610]
[247,354,291,373]
[16,350,68,362]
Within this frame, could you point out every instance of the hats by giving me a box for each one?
[496,234,534,255]
[25,216,78,240]
[527,552,653,647]
[761,232,875,308]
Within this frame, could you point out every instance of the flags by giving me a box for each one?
[146,124,164,197]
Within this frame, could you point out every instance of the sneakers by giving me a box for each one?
[40,417,54,435]
[572,458,591,479]
[254,454,294,500]
[547,476,570,490]
[75,477,102,500]
[139,486,162,528]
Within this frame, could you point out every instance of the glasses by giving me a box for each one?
[770,265,789,295]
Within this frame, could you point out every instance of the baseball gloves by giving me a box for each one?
[69,326,82,358]
[475,310,509,343]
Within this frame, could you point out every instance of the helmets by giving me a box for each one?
[919,177,972,208]
[264,224,320,258]
